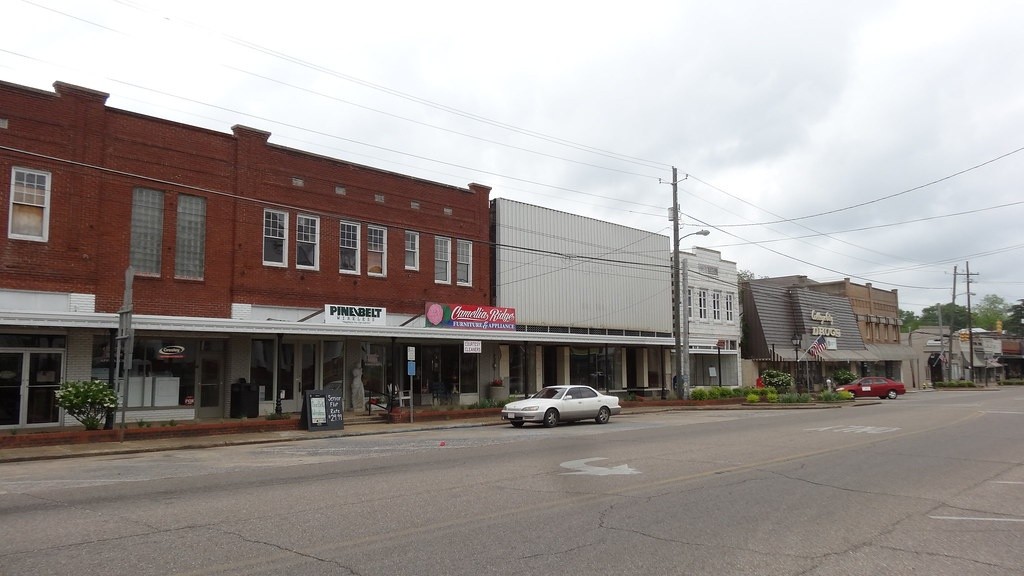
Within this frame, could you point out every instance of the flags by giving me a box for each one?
[812,336,827,356]
[940,352,947,362]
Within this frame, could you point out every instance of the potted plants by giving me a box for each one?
[813,375,824,392]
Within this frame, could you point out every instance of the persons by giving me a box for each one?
[353,363,362,383]
[757,376,764,388]
[826,377,831,392]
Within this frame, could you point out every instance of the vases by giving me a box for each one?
[489,386,502,399]
[366,404,387,411]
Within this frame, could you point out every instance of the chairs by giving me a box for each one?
[387,384,411,407]
[431,382,454,406]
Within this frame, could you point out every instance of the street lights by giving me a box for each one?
[984,356,989,387]
[671,166,710,401]
[791,333,801,395]
[947,265,977,382]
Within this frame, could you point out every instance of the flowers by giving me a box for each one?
[490,377,502,387]
[370,395,385,405]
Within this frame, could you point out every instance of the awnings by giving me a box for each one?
[961,351,984,368]
[771,347,879,361]
[865,344,916,360]
[986,360,1003,368]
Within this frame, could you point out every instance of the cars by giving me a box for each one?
[833,376,906,400]
[322,380,374,397]
[92,359,153,382]
[500,385,621,428]
